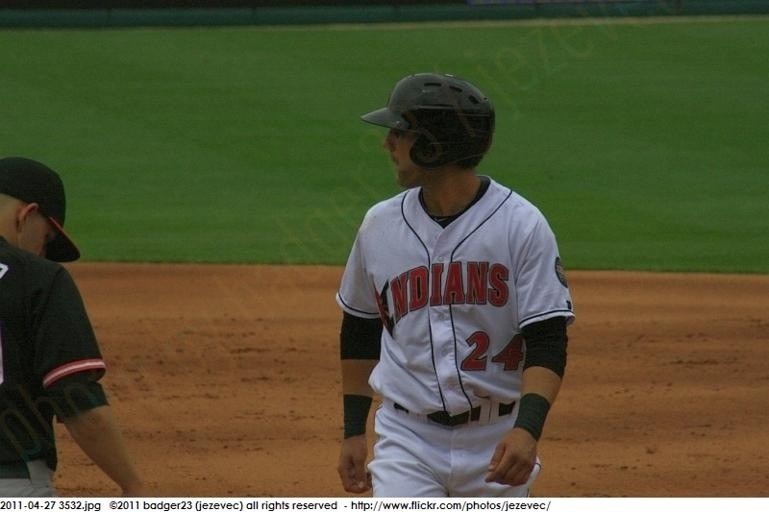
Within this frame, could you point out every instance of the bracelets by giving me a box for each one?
[515,392,550,441]
[342,393,373,439]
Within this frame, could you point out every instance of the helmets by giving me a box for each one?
[359,72,496,170]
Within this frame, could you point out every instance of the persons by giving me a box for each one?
[334,71,577,498]
[1,156,154,498]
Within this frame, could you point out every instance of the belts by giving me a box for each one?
[394,400,516,427]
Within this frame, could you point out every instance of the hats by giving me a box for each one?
[0,156,81,262]
